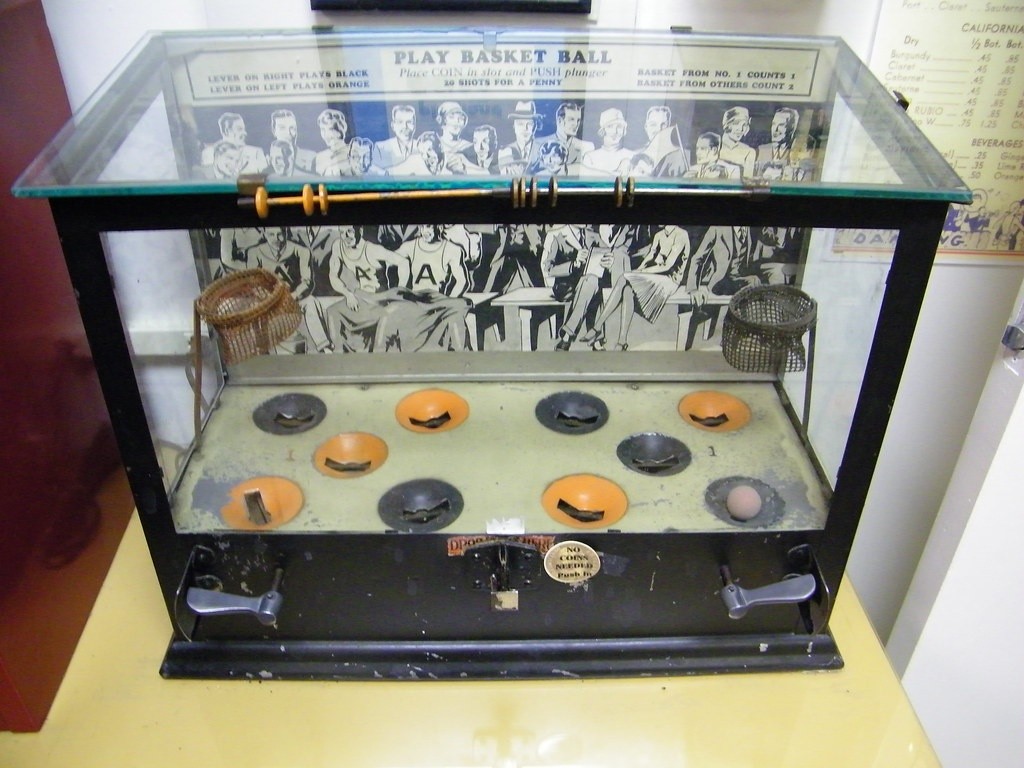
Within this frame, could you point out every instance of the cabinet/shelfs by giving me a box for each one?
[8,26,976,682]
